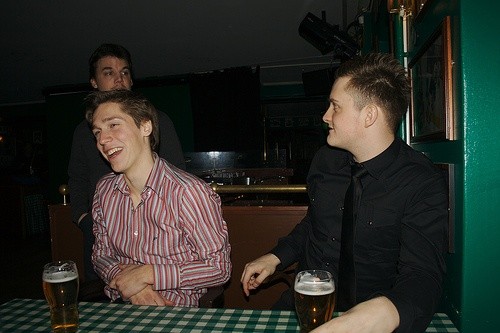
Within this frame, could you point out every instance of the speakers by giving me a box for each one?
[298,12,333,54]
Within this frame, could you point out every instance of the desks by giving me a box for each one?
[0,297,461,333]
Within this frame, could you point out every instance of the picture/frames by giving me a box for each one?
[407,15,458,145]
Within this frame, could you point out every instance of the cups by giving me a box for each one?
[41,260,80,333]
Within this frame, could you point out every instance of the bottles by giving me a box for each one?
[294,270,335,333]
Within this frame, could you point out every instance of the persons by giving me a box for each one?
[84,87,233,309]
[69,41,186,303]
[240,46,453,333]
